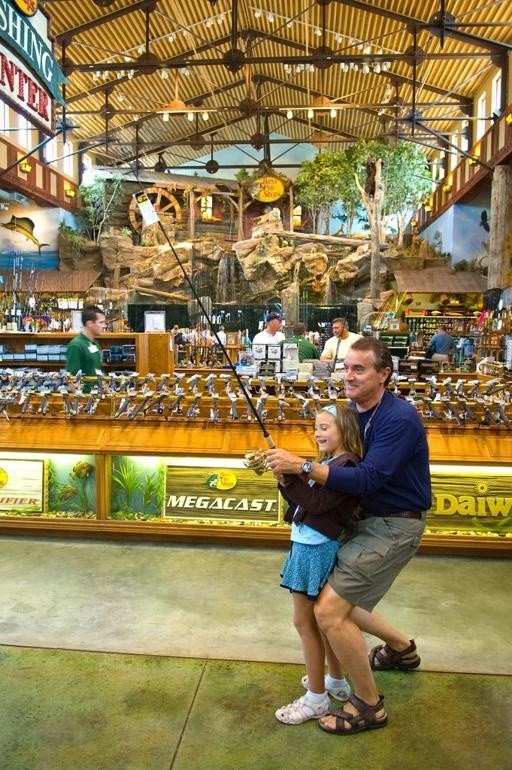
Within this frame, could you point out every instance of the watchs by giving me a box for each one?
[298,457,314,480]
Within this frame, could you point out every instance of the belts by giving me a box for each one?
[367,511,421,519]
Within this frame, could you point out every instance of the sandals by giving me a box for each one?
[318,693,388,736]
[301,673,352,701]
[274,695,332,725]
[369,639,421,670]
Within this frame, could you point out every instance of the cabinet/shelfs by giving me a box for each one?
[0,369,512,465]
[404,315,476,350]
[1,333,174,384]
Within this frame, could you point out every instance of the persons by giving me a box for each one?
[427,324,455,369]
[262,333,435,738]
[172,313,364,375]
[65,305,116,394]
[271,402,368,725]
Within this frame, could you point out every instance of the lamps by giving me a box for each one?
[110,82,393,123]
[92,10,392,81]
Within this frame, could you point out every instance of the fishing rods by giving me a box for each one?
[0,368,512,424]
[329,269,359,375]
[122,151,276,454]
